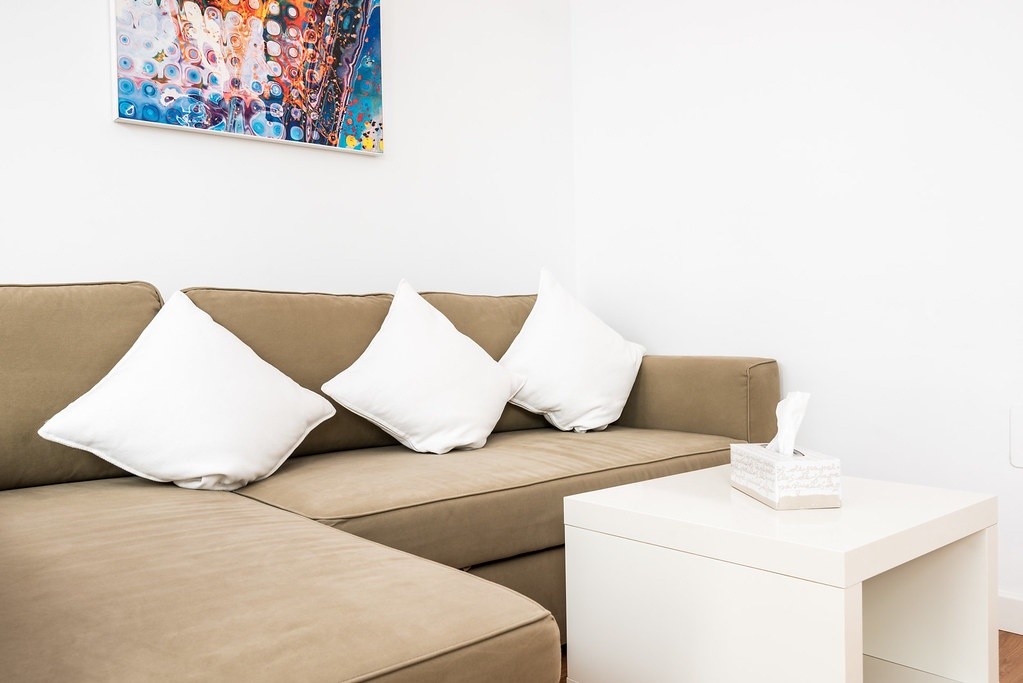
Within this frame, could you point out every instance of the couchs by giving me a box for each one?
[0,281,780,683]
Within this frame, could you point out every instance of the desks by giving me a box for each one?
[564,458,1002,683]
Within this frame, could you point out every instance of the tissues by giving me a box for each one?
[729,390,842,511]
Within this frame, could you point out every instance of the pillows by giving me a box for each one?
[35,288,338,493]
[496,275,645,438]
[319,277,526,456]
